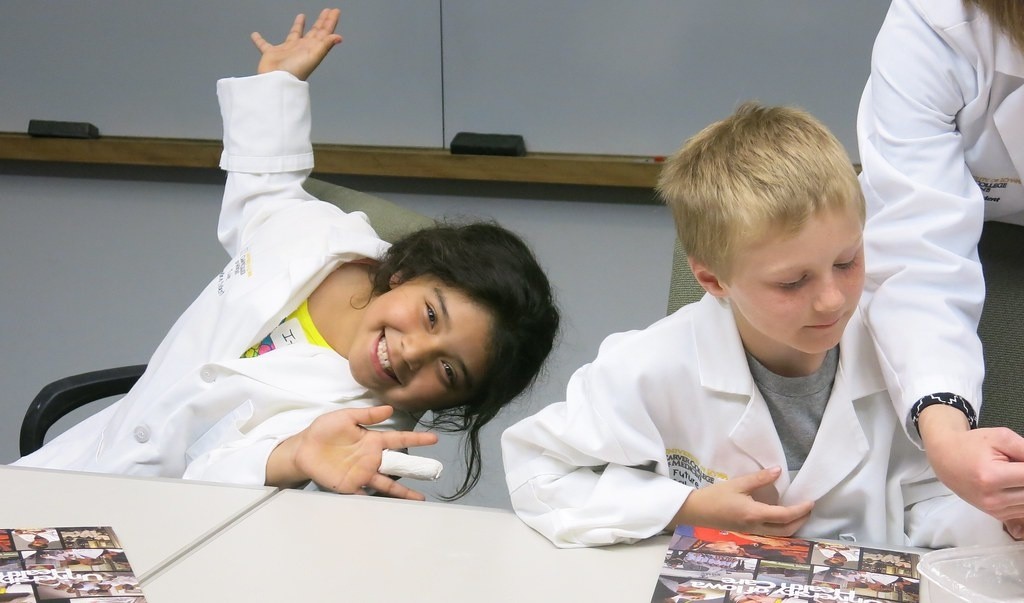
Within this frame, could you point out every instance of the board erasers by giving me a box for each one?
[450,130,526,156]
[27,118,100,139]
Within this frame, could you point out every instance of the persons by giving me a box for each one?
[500,102,1024,550]
[6,8,559,500]
[857,0,1024,521]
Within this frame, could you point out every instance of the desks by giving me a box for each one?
[0,462,1024,603]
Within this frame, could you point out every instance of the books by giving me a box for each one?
[651,525,922,603]
[0,526,146,603]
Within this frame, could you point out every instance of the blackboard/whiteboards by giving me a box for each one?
[0,0,890,195]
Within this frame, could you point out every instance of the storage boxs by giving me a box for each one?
[916,539,1024,603]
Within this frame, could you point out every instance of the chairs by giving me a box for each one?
[18,363,153,457]
